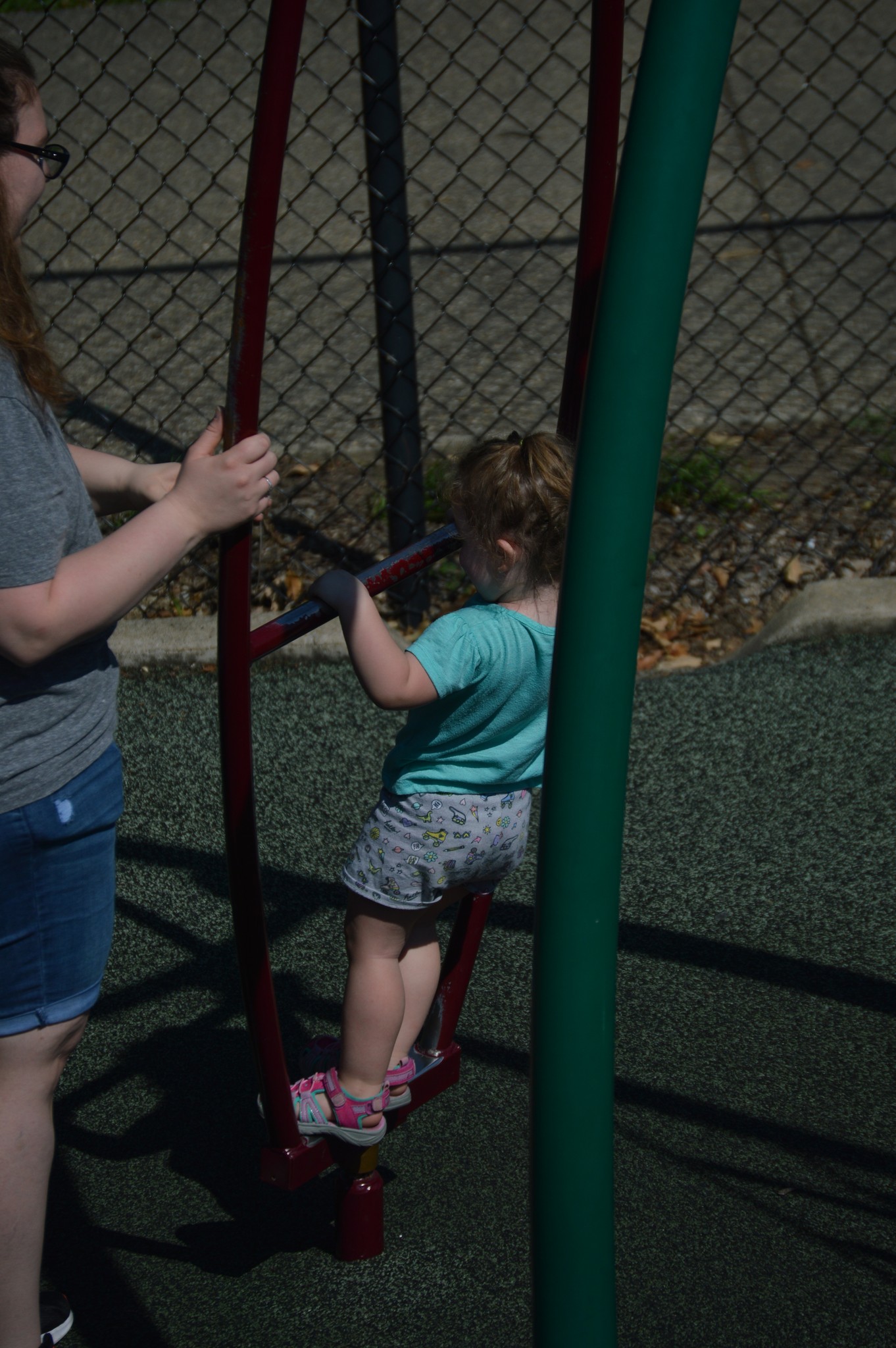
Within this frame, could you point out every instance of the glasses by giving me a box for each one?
[6,140,69,180]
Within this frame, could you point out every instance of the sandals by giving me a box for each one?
[257,1066,391,1147]
[306,1034,414,1111]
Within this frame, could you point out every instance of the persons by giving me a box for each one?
[258,433,582,1140]
[0,45,286,1348]
